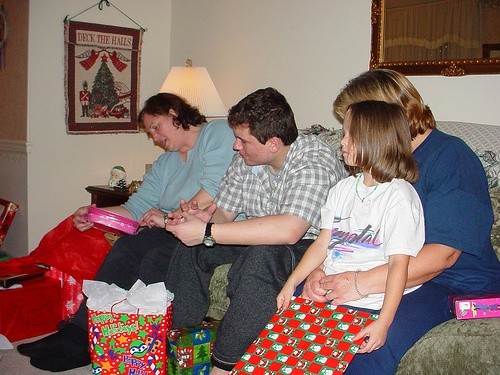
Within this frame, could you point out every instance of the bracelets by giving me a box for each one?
[355,269,368,299]
[164,215,169,226]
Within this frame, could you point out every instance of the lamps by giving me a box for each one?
[158,58,229,118]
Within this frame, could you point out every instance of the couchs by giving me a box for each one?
[205,121,500,375]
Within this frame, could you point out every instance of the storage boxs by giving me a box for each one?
[166,320,220,375]
[447,293,500,321]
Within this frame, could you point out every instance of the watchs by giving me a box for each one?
[203,223,215,247]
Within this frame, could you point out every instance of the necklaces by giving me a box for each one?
[356,171,379,203]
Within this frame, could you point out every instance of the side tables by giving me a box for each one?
[84,184,132,208]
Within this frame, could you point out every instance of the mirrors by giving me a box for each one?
[369,0,500,77]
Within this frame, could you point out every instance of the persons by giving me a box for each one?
[165,87,347,375]
[17,93,248,372]
[276,100,426,353]
[293,68,500,375]
[109,166,127,188]
